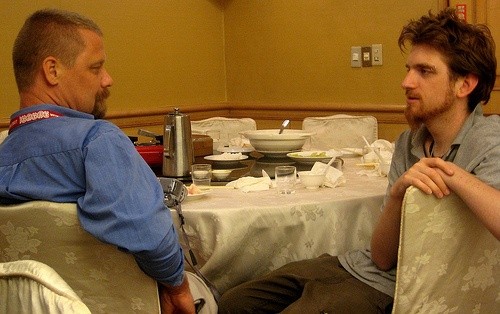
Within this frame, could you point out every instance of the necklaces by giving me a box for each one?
[429,141,445,159]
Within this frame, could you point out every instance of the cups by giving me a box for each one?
[363,146,380,171]
[275,166,296,196]
[192,135,213,163]
[190,163,212,192]
[380,161,392,177]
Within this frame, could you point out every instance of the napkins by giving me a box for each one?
[310,161,346,187]
[223,169,274,193]
[371,139,396,171]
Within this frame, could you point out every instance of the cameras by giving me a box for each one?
[158,178,187,208]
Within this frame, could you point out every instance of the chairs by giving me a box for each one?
[302,115,378,151]
[191,116,256,150]
[391,186,500,314]
[0,201,161,314]
[167,153,389,301]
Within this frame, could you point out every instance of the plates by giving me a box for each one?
[134,146,164,165]
[328,148,361,158]
[203,153,248,164]
[185,185,211,201]
[287,151,340,165]
[217,144,255,154]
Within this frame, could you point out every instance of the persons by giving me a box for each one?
[0,7,218,314]
[216,6,500,314]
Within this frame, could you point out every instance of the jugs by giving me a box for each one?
[164,108,195,183]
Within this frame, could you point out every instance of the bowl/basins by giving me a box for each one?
[298,170,326,189]
[212,169,232,181]
[239,128,312,158]
